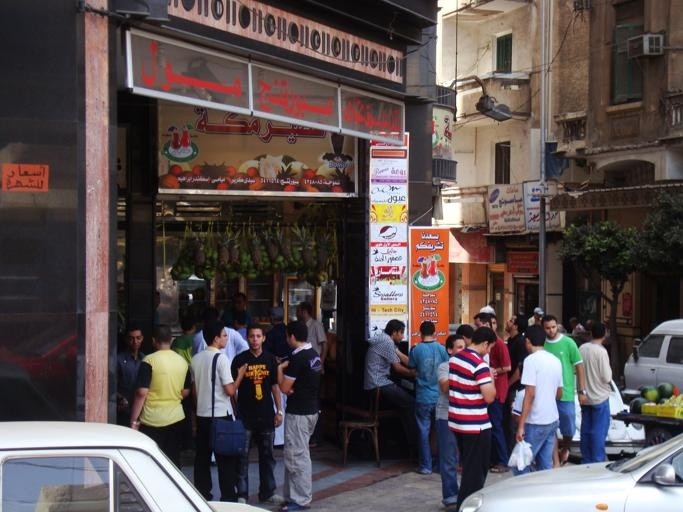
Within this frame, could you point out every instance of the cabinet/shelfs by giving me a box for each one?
[214,282,271,319]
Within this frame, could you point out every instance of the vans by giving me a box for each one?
[618,316,681,394]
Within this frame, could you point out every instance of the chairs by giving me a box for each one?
[338,385,381,469]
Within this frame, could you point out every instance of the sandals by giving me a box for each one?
[490,463,509,473]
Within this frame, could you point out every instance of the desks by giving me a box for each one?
[612,411,682,446]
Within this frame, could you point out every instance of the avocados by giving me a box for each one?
[172,236,337,286]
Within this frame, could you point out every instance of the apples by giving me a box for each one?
[227,166,237,178]
[245,166,258,178]
[193,164,202,177]
[215,181,229,190]
[181,171,196,186]
[330,185,345,193]
[168,164,183,178]
[312,173,327,186]
[299,178,311,191]
[282,183,296,192]
[302,168,315,180]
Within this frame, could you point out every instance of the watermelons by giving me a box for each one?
[630,383,675,414]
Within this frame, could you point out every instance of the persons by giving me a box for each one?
[154,287,328,464]
[116,327,147,428]
[365,301,616,476]
[280,321,322,511]
[232,323,284,506]
[190,319,248,503]
[448,327,497,512]
[130,324,191,471]
[434,334,467,511]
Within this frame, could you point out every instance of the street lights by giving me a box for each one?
[448,73,511,124]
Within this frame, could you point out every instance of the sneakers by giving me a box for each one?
[263,494,291,506]
[238,497,247,504]
[438,503,457,512]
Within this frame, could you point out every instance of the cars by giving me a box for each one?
[457,430,683,511]
[1,366,63,419]
[0,418,256,511]
[511,365,648,463]
[0,319,76,383]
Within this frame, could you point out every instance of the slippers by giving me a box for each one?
[278,502,310,512]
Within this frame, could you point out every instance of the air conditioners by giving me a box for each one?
[574,0,590,10]
[628,34,664,57]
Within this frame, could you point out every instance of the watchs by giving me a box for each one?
[277,410,283,416]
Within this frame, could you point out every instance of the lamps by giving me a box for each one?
[451,76,512,122]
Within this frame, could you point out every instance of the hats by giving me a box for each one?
[534,307,544,315]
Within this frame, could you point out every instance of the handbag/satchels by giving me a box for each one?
[209,419,247,458]
